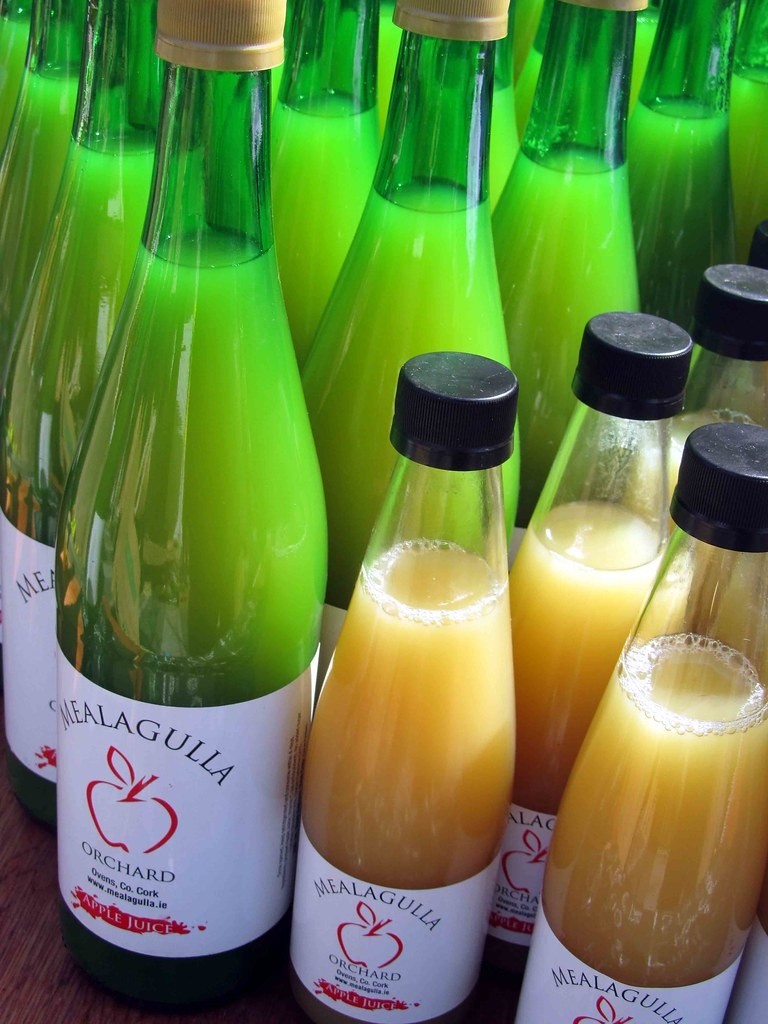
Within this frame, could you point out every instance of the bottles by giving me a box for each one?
[1,1,768,1024]
